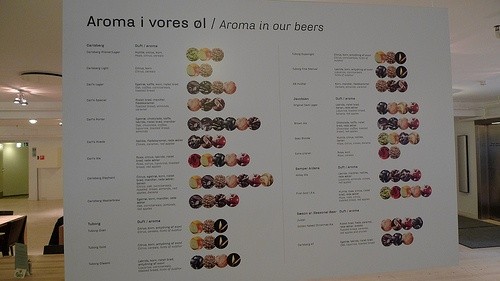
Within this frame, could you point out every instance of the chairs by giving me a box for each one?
[0,210,14,216]
[1,216,27,256]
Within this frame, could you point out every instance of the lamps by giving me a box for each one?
[13,89,29,107]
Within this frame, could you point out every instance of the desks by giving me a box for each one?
[0,214,28,256]
[0,253,66,281]
